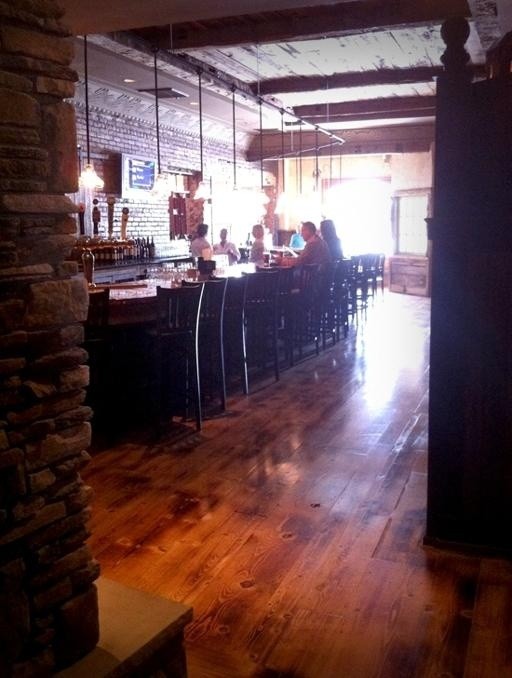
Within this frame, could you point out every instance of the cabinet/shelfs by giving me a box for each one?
[169,195,205,236]
[385,254,432,297]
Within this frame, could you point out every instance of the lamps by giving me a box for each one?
[192,71,217,201]
[222,91,246,204]
[275,110,297,216]
[151,51,176,199]
[78,31,107,190]
[248,99,271,207]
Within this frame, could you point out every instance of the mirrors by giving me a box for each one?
[390,187,433,255]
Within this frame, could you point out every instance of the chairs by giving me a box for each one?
[86,250,386,442]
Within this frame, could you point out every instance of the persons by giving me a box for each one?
[277,220,331,333]
[244,223,267,264]
[320,220,344,273]
[190,223,212,261]
[212,228,242,266]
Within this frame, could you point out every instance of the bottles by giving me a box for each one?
[237,241,253,259]
[147,258,200,283]
[81,247,95,285]
[262,252,272,266]
[77,235,157,265]
